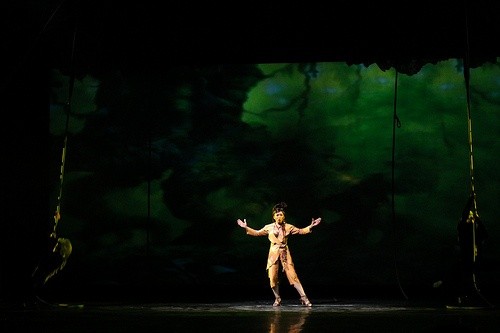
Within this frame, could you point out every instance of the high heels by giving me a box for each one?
[300,295,312,307]
[272,297,282,307]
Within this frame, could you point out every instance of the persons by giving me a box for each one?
[237,202,322,307]
